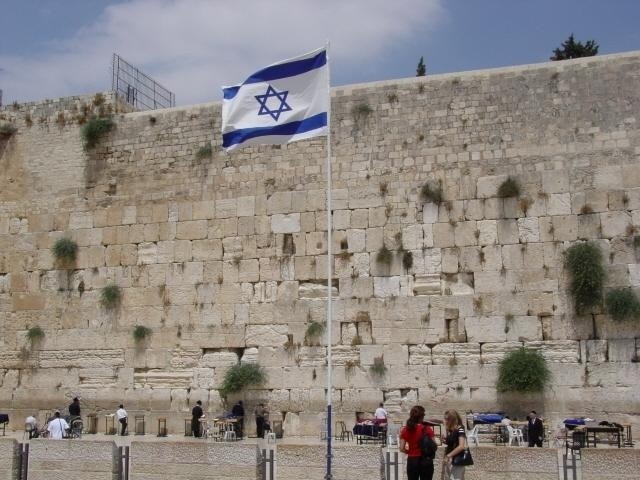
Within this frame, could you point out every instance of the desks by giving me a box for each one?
[0,413,9,436]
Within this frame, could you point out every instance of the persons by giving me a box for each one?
[25,412,38,437]
[69,396,80,433]
[255,404,269,438]
[375,403,388,446]
[439,408,467,480]
[526,411,544,447]
[400,405,435,479]
[500,414,511,423]
[191,400,203,438]
[115,404,129,436]
[47,411,69,439]
[232,401,243,437]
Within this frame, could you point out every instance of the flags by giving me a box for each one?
[221,46,328,155]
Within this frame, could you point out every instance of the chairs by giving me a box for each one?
[23,411,84,441]
[320,410,634,449]
[191,414,240,442]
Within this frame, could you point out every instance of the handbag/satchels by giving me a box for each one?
[420,434,437,459]
[450,446,474,466]
